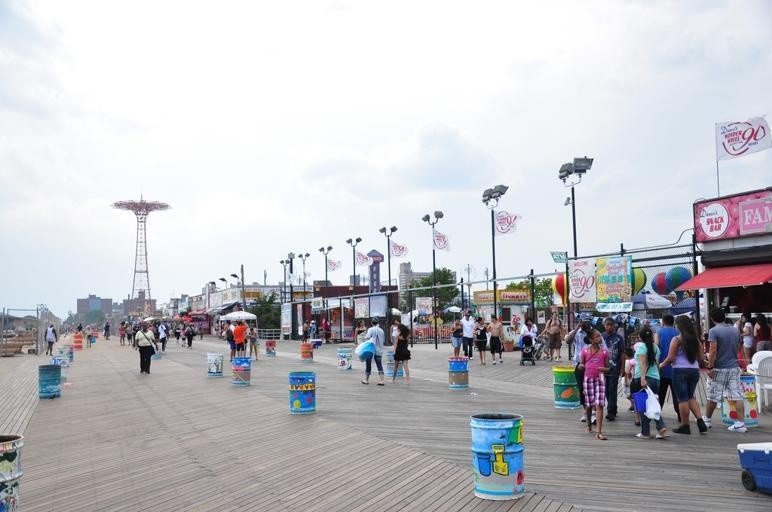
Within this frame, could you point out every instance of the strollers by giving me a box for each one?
[519,334,536,366]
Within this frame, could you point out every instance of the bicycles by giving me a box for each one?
[534,333,552,361]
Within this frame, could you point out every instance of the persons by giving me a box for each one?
[45,324,57,355]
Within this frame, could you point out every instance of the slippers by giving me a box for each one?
[635,432,649,440]
[360,379,413,386]
[656,432,671,440]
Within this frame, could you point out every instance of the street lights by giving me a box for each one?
[280,259,290,303]
[229,273,240,302]
[346,235,363,341]
[421,209,444,351]
[219,277,228,302]
[297,253,310,302]
[379,226,399,347]
[558,155,595,316]
[318,245,333,320]
[210,281,217,308]
[483,182,510,319]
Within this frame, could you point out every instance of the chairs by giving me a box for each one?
[746,350,772,414]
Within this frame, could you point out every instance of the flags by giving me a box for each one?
[715,114,771,161]
[355,252,373,266]
[327,259,338,270]
[433,229,448,250]
[493,209,520,236]
[389,240,408,256]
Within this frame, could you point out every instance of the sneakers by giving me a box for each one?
[699,423,707,433]
[580,410,587,422]
[672,426,691,435]
[702,416,712,428]
[140,369,151,375]
[605,412,618,419]
[727,424,749,434]
[590,411,596,425]
[608,411,615,422]
[463,356,504,366]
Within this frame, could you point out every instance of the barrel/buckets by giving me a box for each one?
[300,344,313,361]
[471,413,524,501]
[39,334,83,400]
[385,351,403,378]
[266,340,276,357]
[288,371,316,415]
[720,375,761,429]
[93,330,98,338]
[337,348,352,369]
[0,433,24,512]
[448,357,469,390]
[553,368,581,408]
[207,353,223,378]
[231,357,250,388]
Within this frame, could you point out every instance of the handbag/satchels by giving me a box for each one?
[151,347,155,356]
[354,338,376,362]
[90,337,96,343]
[632,392,659,413]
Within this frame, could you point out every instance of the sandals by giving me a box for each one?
[595,433,608,440]
[636,419,640,427]
[586,425,593,434]
